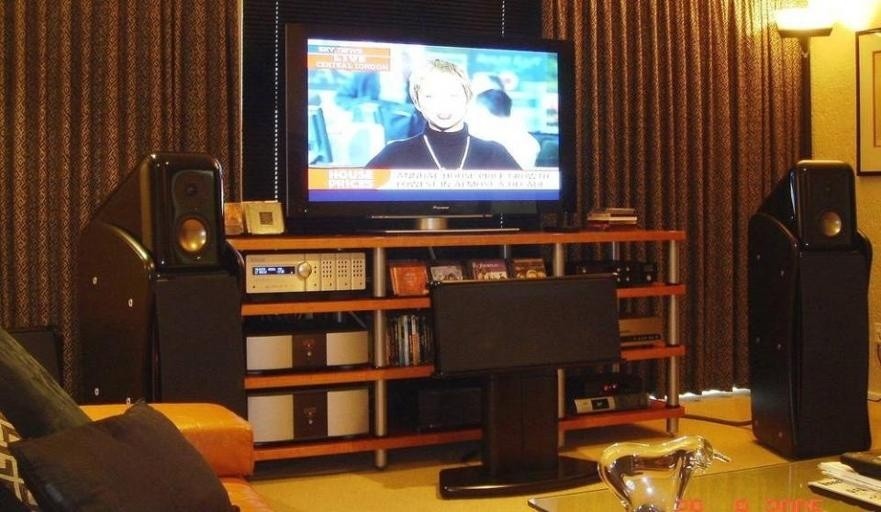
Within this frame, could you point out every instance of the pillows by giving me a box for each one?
[0,328,91,439]
[7,399,240,511]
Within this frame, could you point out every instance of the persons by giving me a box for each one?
[332,46,542,170]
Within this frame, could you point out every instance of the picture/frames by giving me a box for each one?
[856,27,881,176]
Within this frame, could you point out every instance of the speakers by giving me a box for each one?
[747,159,874,462]
[82,150,248,422]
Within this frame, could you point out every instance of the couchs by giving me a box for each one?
[0,326,272,511]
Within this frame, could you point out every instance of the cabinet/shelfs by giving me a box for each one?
[225,229,687,469]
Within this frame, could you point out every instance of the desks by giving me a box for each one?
[528,454,881,512]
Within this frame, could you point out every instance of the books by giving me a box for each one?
[586,207,639,231]
[384,313,436,367]
[385,258,547,297]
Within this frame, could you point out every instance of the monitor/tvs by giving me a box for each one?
[285,22,577,234]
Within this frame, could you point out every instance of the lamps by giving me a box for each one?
[774,8,833,159]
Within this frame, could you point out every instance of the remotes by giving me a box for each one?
[839,451,881,480]
[807,478,881,512]
